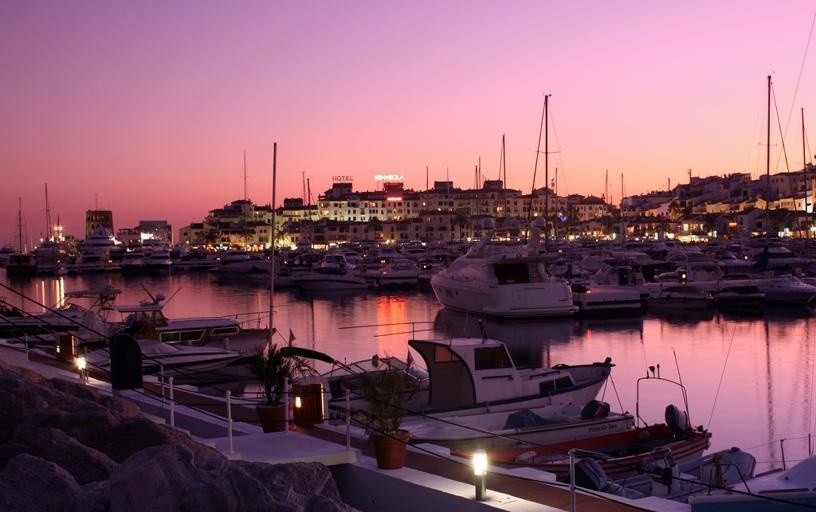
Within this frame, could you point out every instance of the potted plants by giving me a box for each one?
[357,367,422,470]
[234,342,318,433]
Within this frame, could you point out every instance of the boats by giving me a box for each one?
[0,303,276,385]
